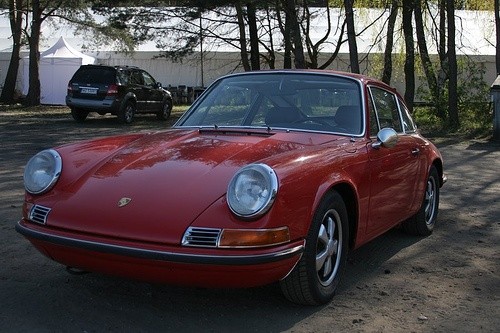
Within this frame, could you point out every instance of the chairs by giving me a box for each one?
[264,107,304,128]
[332,105,362,135]
[132,73,145,85]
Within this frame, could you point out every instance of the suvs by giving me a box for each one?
[65,62,173,125]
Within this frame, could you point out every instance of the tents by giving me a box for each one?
[20,36,95,105]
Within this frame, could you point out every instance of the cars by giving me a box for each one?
[15,69,448,301]
